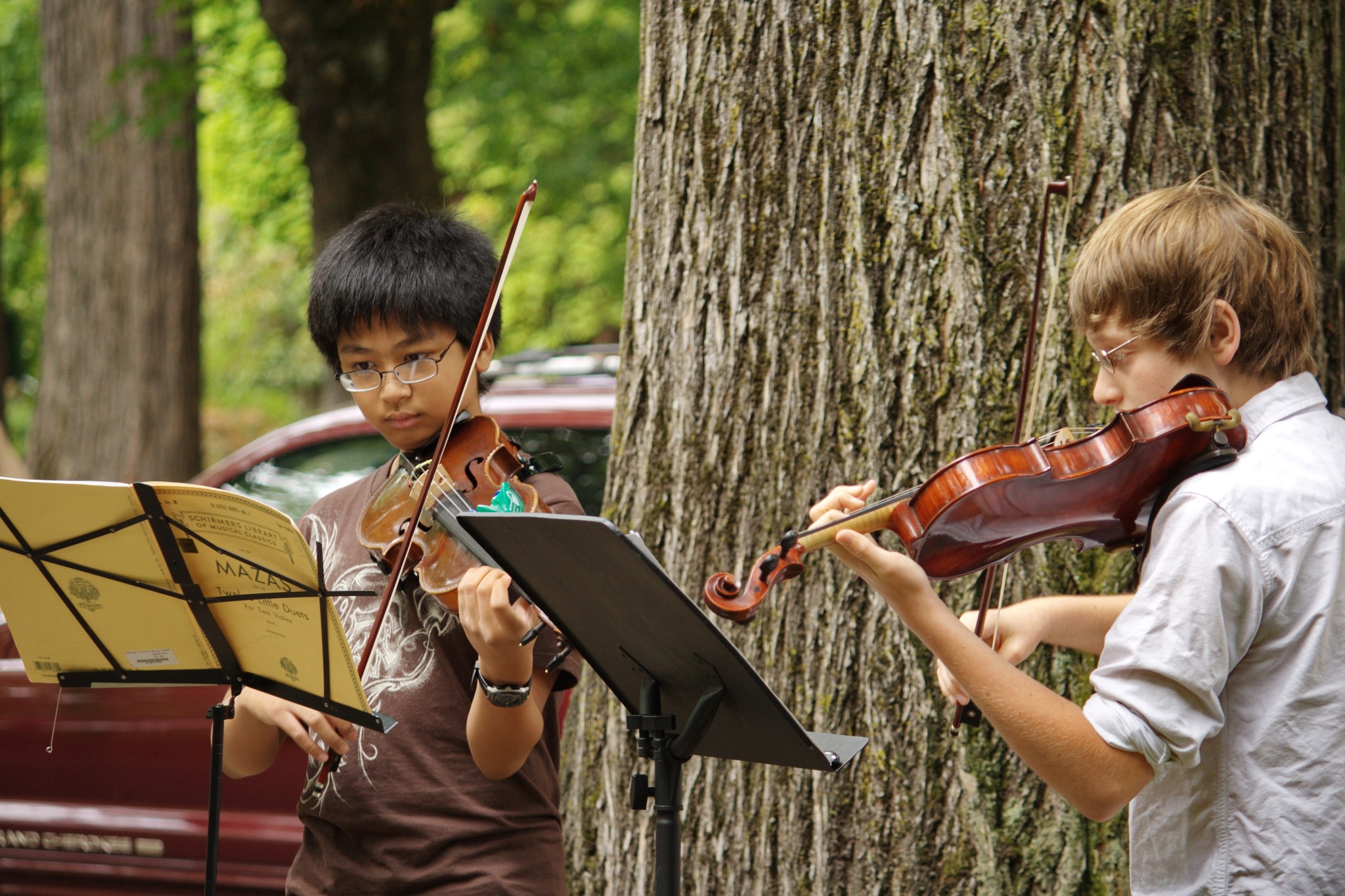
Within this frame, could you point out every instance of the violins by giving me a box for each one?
[357,412,580,675]
[704,386,1248,619]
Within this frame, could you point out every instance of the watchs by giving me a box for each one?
[469,659,533,708]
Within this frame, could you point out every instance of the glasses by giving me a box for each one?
[1091,333,1145,375]
[332,334,461,394]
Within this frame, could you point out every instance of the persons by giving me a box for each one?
[806,181,1344,896]
[212,201,586,895]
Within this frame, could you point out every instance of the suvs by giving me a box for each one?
[0,341,626,896]
